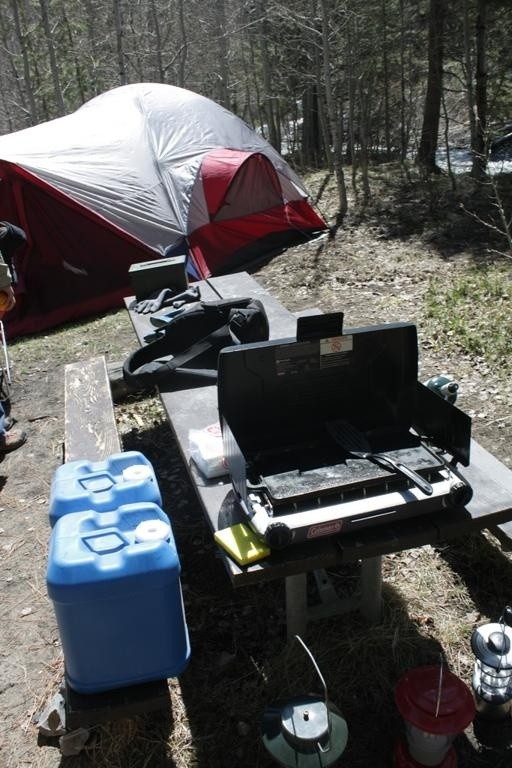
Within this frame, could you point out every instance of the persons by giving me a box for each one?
[0,250,28,454]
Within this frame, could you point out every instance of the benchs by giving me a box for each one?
[64,356,120,466]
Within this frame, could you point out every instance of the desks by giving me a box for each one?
[123,270,512,645]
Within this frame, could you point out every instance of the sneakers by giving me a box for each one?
[3,415,15,430]
[1,427,28,452]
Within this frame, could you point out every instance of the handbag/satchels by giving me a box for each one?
[119,296,271,392]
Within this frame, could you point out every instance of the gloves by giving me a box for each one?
[133,287,173,314]
[162,285,201,309]
